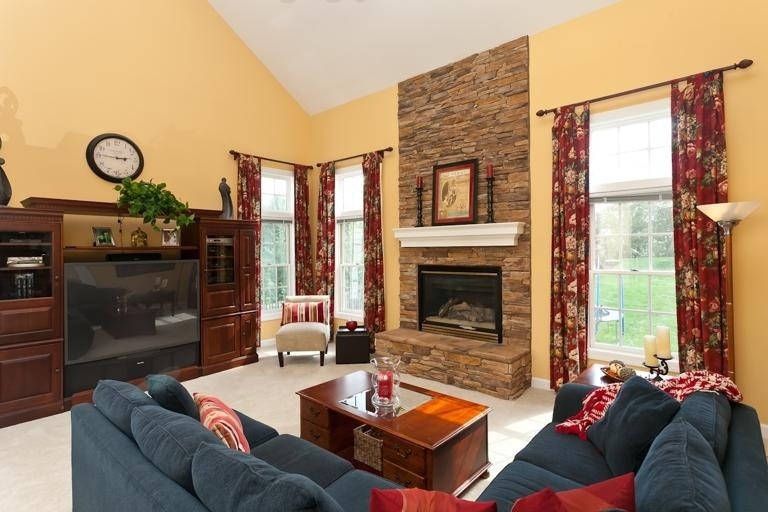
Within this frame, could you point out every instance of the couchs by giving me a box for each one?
[468,382,768,512]
[71,394,429,510]
[275,292,333,369]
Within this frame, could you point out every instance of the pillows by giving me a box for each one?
[91,377,156,439]
[145,373,200,420]
[129,405,222,486]
[368,487,497,505]
[583,373,681,481]
[512,472,638,512]
[196,392,253,457]
[635,412,733,512]
[191,440,343,511]
[662,388,731,470]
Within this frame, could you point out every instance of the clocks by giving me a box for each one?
[84,131,145,183]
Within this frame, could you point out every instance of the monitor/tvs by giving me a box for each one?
[64,259,200,399]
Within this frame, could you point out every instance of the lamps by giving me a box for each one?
[694,199,760,392]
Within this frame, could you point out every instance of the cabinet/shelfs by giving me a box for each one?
[0,207,62,431]
[198,219,261,373]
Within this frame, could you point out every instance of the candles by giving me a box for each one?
[415,173,424,192]
[655,325,672,363]
[485,162,495,180]
[643,334,663,370]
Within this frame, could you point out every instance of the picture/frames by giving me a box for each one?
[430,158,477,227]
